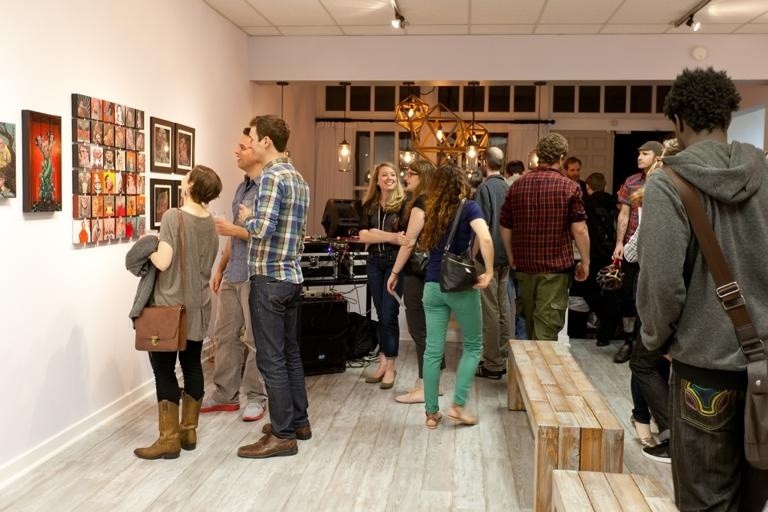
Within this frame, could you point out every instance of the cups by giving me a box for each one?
[212,212,226,224]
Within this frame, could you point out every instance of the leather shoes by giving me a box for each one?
[630,414,657,448]
[238,431,297,458]
[365,371,385,383]
[262,423,312,439]
[394,387,443,403]
[380,370,396,388]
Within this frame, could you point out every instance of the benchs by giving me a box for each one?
[507,338,682,511]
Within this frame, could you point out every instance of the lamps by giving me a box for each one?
[686,16,700,32]
[527,81,547,170]
[336,82,352,173]
[391,14,405,30]
[392,80,490,176]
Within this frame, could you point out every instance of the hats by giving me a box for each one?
[638,141,664,156]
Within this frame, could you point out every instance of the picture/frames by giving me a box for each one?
[150,115,196,230]
[21,108,62,213]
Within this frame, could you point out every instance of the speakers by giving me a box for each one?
[299,292,347,376]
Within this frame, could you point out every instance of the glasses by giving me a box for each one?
[407,171,418,178]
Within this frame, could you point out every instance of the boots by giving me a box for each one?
[133,400,182,459]
[179,388,205,450]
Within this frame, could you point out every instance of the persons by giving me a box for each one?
[76,98,146,244]
[178,132,193,168]
[635,66,768,511]
[358,161,494,428]
[587,139,680,461]
[235,113,311,458]
[35,129,60,207]
[157,128,171,164]
[132,163,224,458]
[475,132,592,379]
[197,128,266,421]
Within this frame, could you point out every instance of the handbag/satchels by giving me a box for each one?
[743,359,768,471]
[134,304,187,353]
[596,265,626,291]
[440,250,478,293]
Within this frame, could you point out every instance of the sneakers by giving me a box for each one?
[242,400,267,421]
[476,361,506,379]
[641,439,672,463]
[199,395,240,413]
[581,321,610,346]
[614,344,633,363]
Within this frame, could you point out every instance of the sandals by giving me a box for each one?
[426,411,442,428]
[446,404,479,425]
